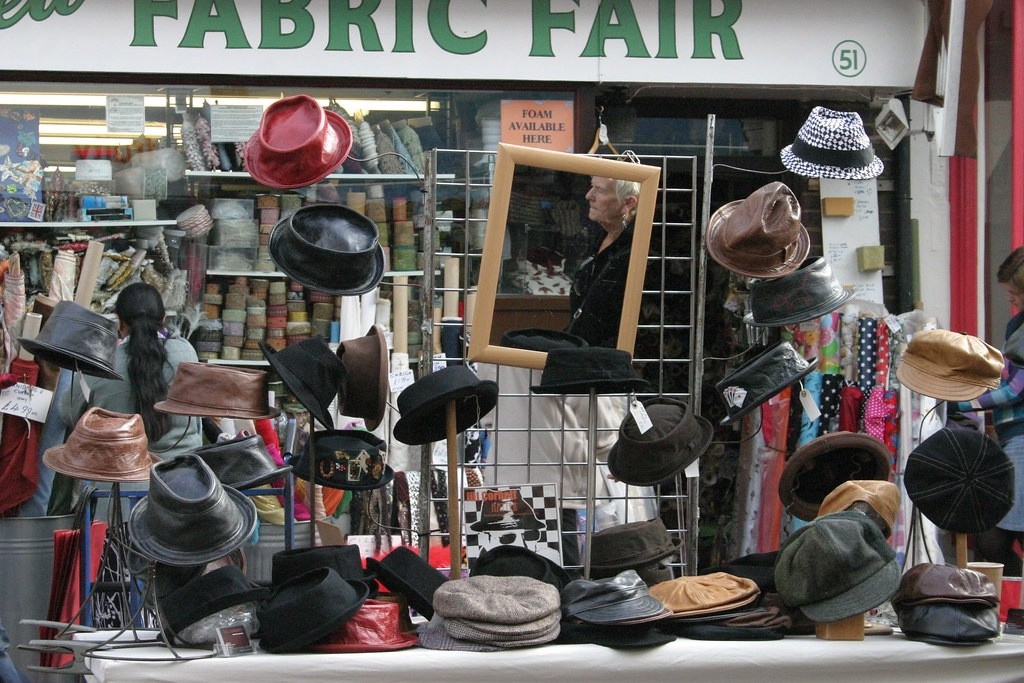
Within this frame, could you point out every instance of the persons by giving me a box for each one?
[58,283,203,516]
[947,246,1024,577]
[563,175,641,349]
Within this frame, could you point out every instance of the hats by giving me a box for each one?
[581,516,686,570]
[817,479,901,535]
[184,432,294,490]
[780,105,885,180]
[336,324,389,432]
[257,336,349,429]
[18,298,125,381]
[777,430,892,522]
[904,427,1014,534]
[153,360,282,420]
[126,452,259,569]
[42,406,166,484]
[704,179,810,280]
[715,341,820,426]
[393,365,499,444]
[125,543,1003,650]
[530,348,652,395]
[244,94,353,190]
[771,510,901,622]
[607,398,714,488]
[896,328,1005,402]
[290,429,396,492]
[740,255,859,327]
[267,203,386,296]
[501,327,589,353]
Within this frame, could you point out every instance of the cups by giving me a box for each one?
[966,561,1004,616]
[999,576,1023,622]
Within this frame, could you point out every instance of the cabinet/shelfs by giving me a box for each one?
[180,172,459,365]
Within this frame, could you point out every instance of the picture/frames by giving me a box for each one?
[462,482,564,571]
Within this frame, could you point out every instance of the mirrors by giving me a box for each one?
[469,142,663,376]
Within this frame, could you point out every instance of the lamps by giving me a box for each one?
[0,81,444,146]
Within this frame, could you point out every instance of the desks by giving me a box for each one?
[65,625,1023,683]
